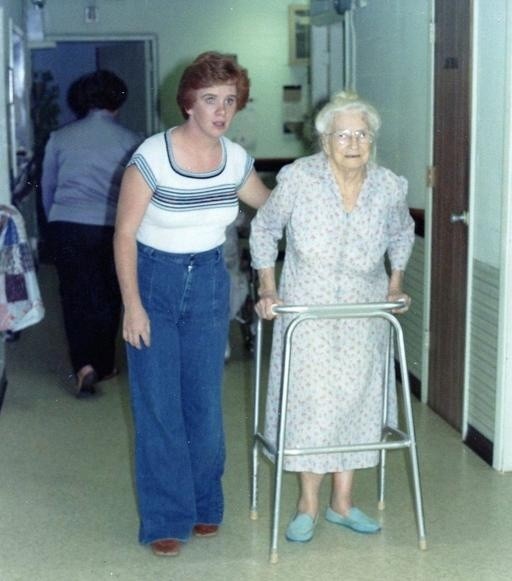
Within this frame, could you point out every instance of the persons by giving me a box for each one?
[41,70,146,398]
[249,92,415,543]
[112,51,271,556]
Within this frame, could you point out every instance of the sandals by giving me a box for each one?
[76,366,119,396]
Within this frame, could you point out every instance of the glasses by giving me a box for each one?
[325,128,374,144]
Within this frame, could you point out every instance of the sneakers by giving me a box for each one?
[152,540,179,555]
[325,503,381,533]
[285,511,319,542]
[193,524,218,536]
